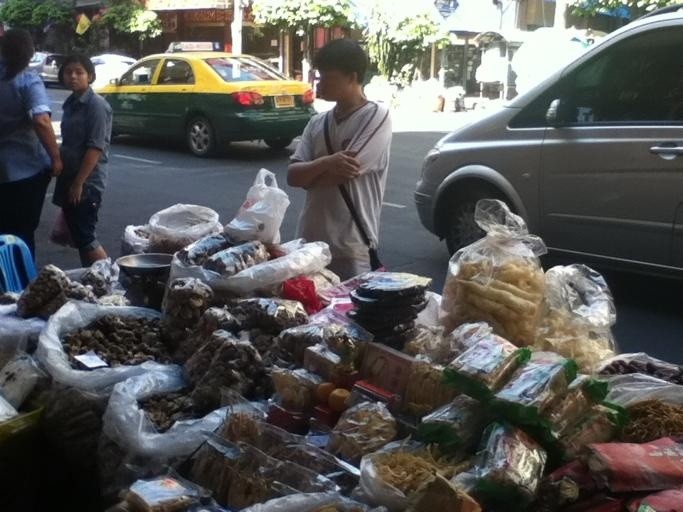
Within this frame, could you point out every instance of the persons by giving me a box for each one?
[284,38,392,284]
[0,28,64,295]
[50,55,112,267]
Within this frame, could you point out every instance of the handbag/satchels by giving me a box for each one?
[52,178,97,212]
[369,246,389,271]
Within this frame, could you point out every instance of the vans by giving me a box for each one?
[414,4,682,285]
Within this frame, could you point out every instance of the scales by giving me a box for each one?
[116,253,174,312]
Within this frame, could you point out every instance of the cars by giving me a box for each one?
[28,52,151,91]
[94,41,320,157]
